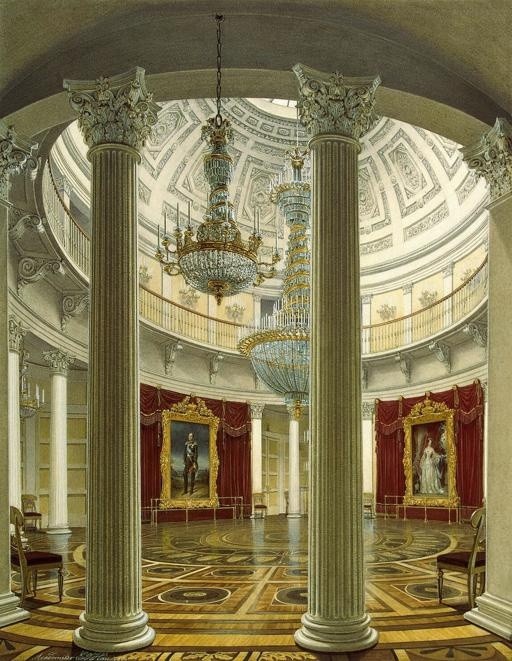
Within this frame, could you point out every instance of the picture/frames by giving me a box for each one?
[401,399,462,509]
[159,395,221,509]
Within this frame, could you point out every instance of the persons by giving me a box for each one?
[418,437,447,495]
[181,432,199,496]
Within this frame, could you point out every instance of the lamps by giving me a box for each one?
[236,99,312,421]
[155,14,282,309]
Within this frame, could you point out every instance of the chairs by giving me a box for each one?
[9,506,64,608]
[253,493,267,519]
[363,493,375,520]
[435,506,486,610]
[20,494,42,533]
